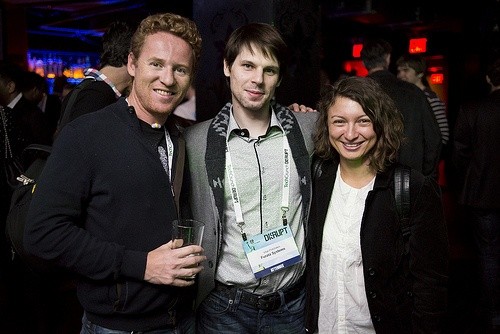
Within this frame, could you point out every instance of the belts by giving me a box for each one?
[216,279,306,312]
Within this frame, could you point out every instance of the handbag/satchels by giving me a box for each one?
[6,79,96,265]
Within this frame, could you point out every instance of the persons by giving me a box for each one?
[0,61,75,230]
[24,13,318,334]
[361,38,451,188]
[57,18,142,127]
[184,22,321,334]
[306,76,448,334]
[453,60,500,334]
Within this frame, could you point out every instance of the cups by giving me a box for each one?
[169,219,204,279]
